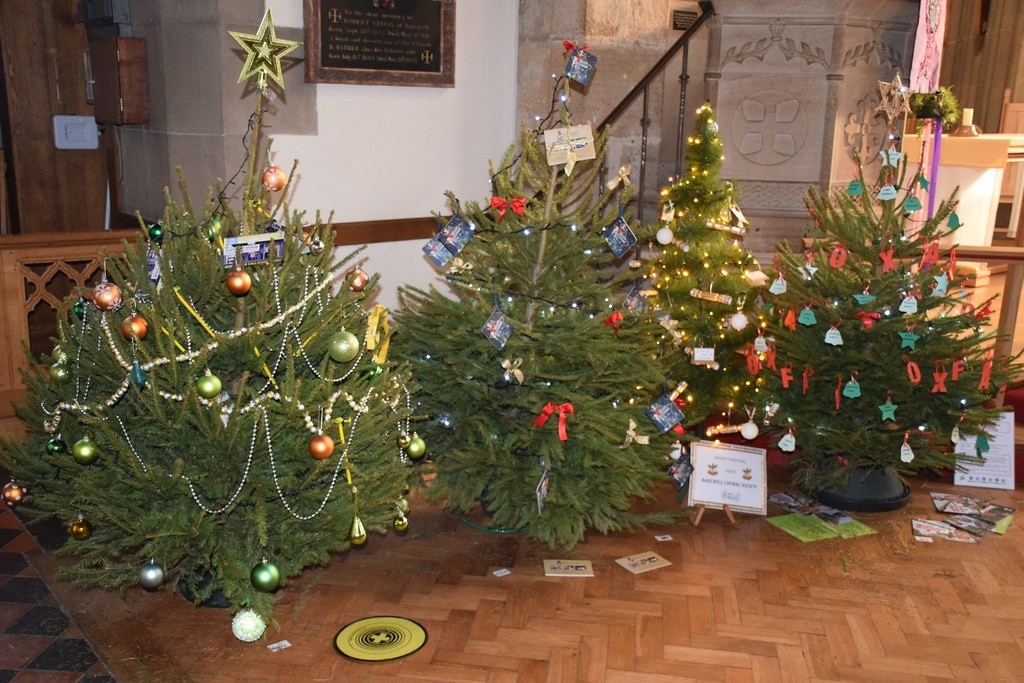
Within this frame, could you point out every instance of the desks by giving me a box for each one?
[980,134,1024,239]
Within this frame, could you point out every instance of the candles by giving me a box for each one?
[962,106,975,126]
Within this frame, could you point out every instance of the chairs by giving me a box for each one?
[1000,87,1024,205]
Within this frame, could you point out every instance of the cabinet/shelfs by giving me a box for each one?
[88,38,150,126]
